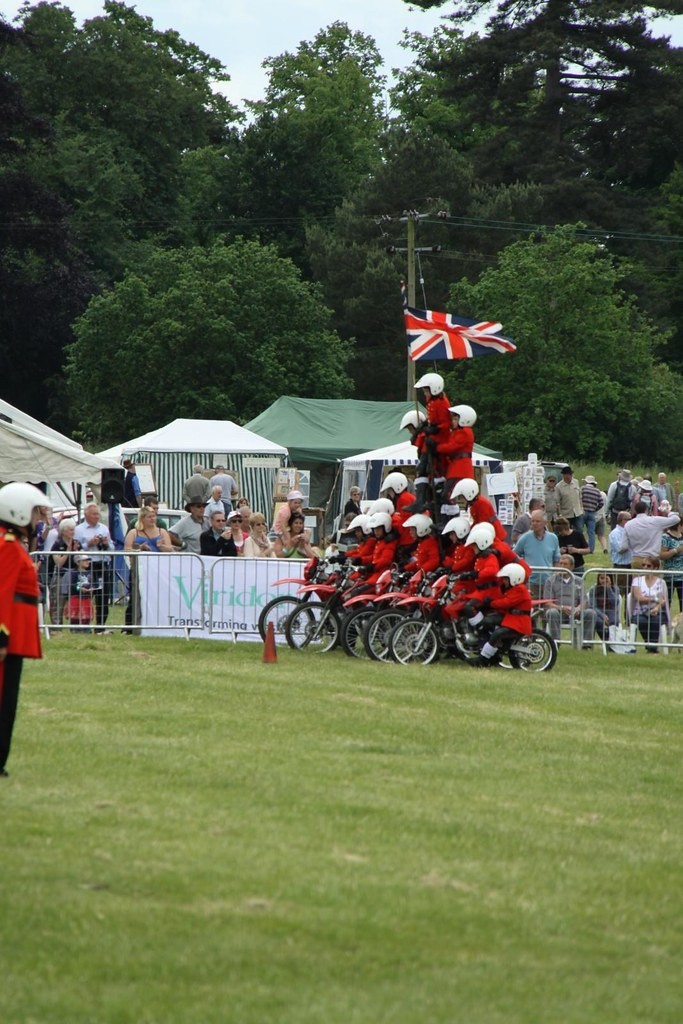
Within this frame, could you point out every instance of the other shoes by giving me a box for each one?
[603,549,607,553]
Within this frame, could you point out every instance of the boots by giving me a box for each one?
[465,641,498,665]
[400,476,447,535]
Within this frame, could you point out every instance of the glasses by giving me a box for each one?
[549,480,556,482]
[641,563,651,567]
[256,522,266,526]
[230,519,243,523]
[213,519,227,522]
[196,505,206,508]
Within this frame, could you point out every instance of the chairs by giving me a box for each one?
[540,592,675,654]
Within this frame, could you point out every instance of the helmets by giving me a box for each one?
[345,498,395,534]
[496,562,526,586]
[414,372,444,396]
[448,404,477,426]
[0,482,51,527]
[378,471,407,495]
[402,478,496,551]
[399,409,425,430]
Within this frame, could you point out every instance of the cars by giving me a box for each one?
[46,505,191,529]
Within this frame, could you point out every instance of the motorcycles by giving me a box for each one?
[258,550,560,671]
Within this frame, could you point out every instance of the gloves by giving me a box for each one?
[410,421,441,454]
[326,550,498,590]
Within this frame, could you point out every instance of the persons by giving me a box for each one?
[0,373,683,778]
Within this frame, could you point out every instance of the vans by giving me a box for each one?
[502,461,569,502]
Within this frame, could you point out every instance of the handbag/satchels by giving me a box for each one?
[608,623,636,653]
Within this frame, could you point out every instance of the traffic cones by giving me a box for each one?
[262,620,279,664]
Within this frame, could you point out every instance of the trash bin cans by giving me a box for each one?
[301,506,326,549]
[542,462,570,485]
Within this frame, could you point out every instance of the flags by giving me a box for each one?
[400,281,517,361]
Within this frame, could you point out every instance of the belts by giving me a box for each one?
[489,515,498,523]
[509,609,531,614]
[476,581,499,591]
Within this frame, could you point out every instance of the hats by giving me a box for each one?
[184,495,208,513]
[286,490,307,500]
[560,467,652,491]
[228,510,240,520]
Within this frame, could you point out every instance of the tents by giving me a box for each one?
[93,419,290,530]
[0,398,83,450]
[341,438,504,520]
[240,395,429,535]
[0,419,126,511]
